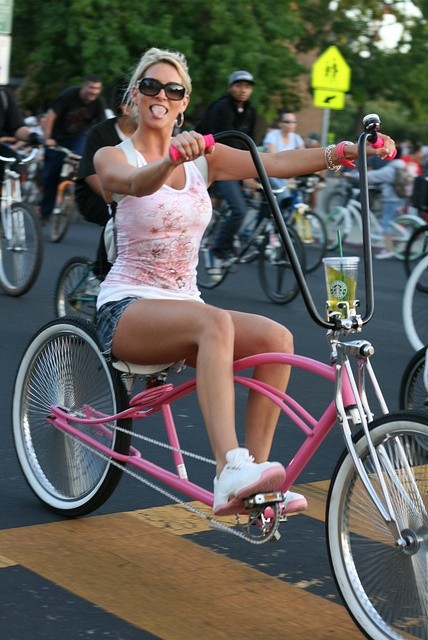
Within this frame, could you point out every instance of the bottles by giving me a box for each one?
[228,71,256,86]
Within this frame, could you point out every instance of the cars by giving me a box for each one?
[323,255,359,320]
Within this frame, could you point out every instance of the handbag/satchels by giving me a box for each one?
[324,143,341,170]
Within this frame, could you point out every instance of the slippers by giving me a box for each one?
[389,165,414,198]
[415,176,427,211]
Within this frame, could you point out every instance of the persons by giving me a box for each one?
[344,146,407,259]
[95,45,399,522]
[402,143,423,178]
[262,106,308,205]
[193,70,258,277]
[38,75,107,224]
[0,88,45,206]
[75,81,140,296]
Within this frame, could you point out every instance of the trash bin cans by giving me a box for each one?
[336,138,358,170]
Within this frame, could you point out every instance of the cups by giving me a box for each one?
[282,121,298,123]
[137,77,185,100]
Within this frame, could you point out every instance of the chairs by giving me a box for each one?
[375,250,393,260]
[203,250,222,280]
[282,491,307,514]
[213,448,285,516]
